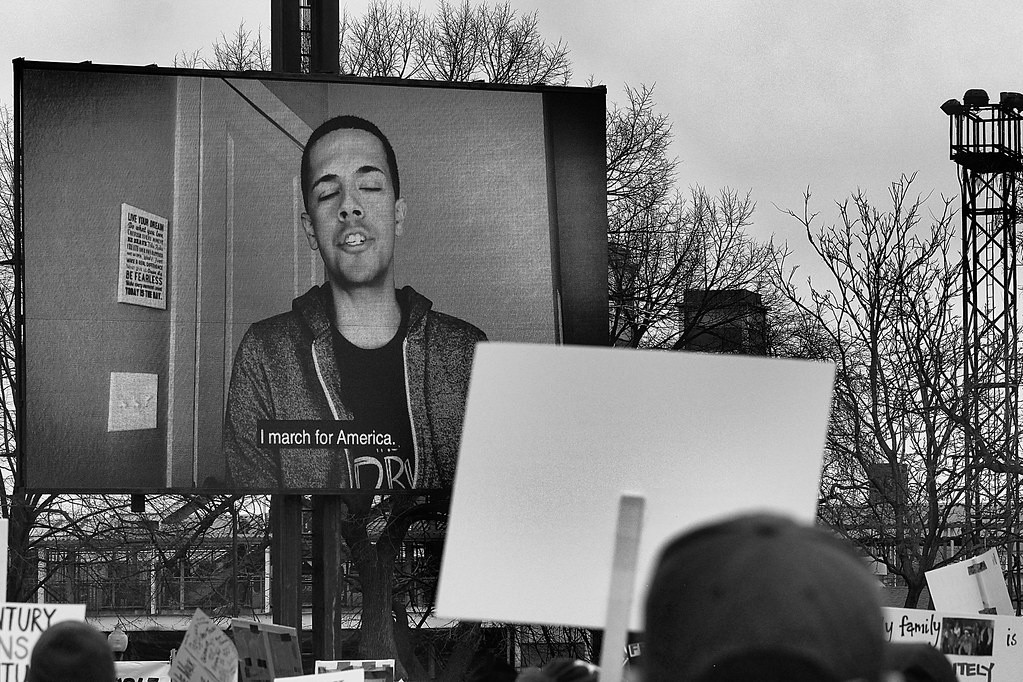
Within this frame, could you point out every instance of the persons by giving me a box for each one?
[24,620,118,682]
[943,618,993,655]
[886,642,959,681]
[624,511,885,682]
[222,116,486,491]
